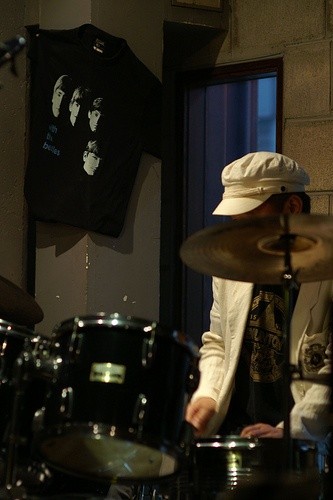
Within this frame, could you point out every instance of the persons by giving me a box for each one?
[183,151,333,442]
[29,74,115,186]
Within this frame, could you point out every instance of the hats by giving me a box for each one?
[212,152,311,215]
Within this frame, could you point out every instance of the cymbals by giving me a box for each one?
[180,211,333,285]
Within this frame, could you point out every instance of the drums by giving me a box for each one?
[197,430,322,500]
[1,321,69,406]
[32,313,197,482]
